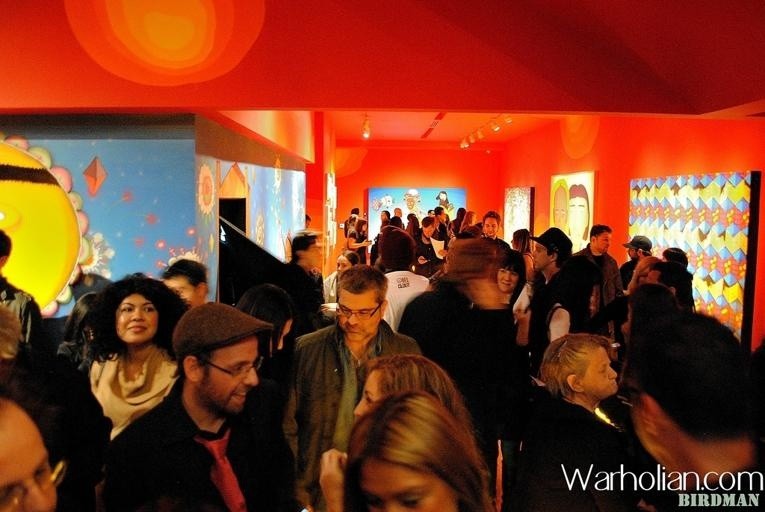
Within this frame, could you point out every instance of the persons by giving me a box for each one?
[1,206,765,510]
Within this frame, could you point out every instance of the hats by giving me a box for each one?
[529,227,573,259]
[623,236,652,255]
[173,302,275,355]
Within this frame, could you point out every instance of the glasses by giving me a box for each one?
[203,355,263,378]
[0,460,67,510]
[335,303,382,320]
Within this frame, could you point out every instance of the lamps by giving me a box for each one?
[461,112,518,153]
[360,109,373,142]
